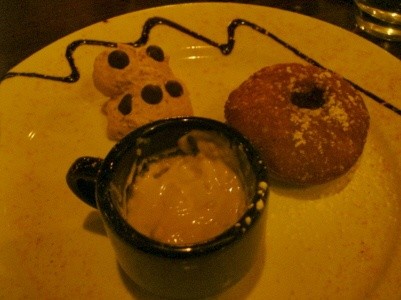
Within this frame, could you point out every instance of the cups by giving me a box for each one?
[66,116,269,300]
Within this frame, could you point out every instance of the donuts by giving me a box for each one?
[223,62,371,189]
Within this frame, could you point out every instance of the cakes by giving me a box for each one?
[92,43,194,142]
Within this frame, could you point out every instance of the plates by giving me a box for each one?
[0,1,400,299]
